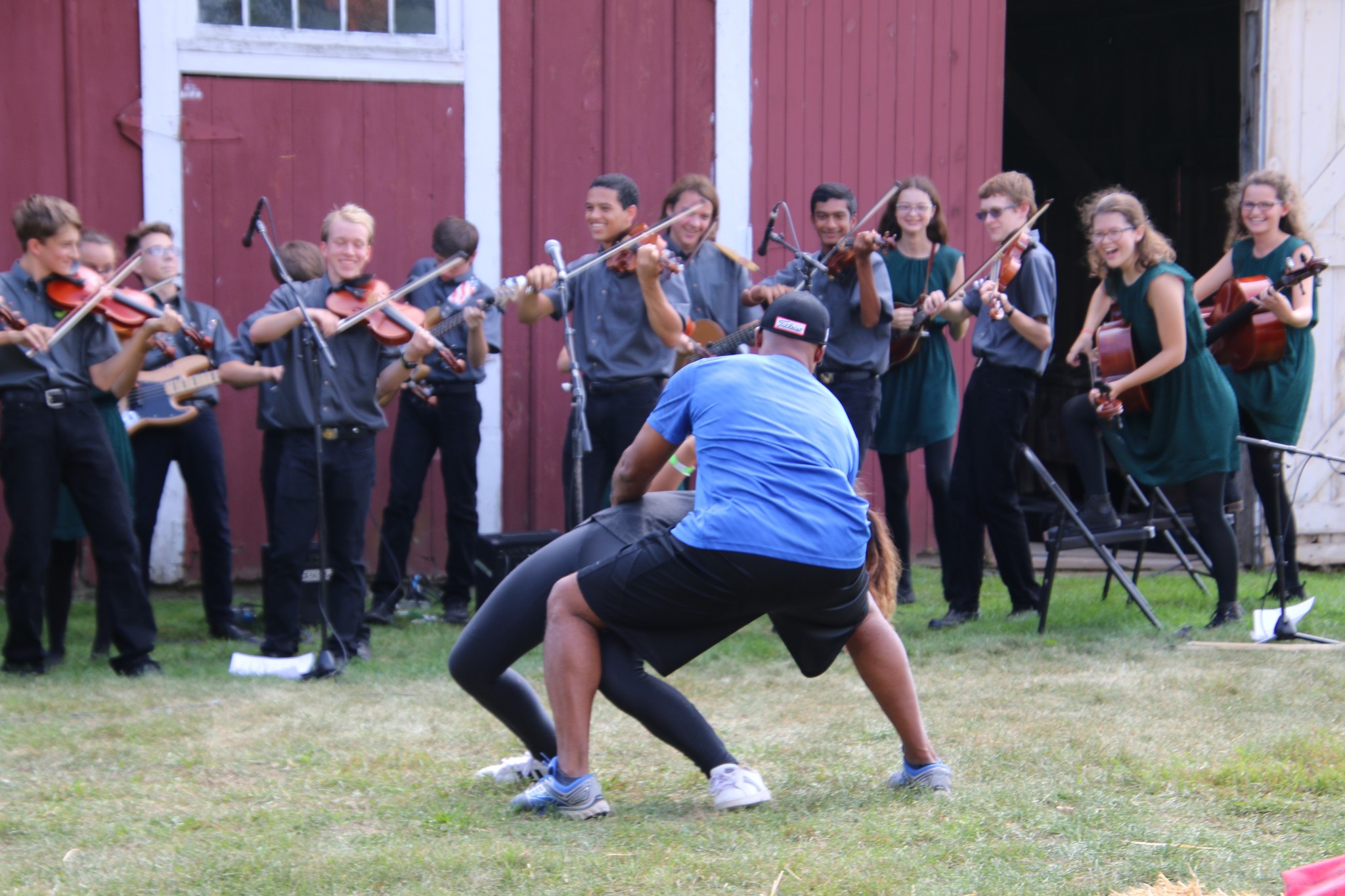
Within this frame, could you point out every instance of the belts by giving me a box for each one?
[586,377,655,392]
[413,382,472,395]
[293,425,364,441]
[2,386,94,408]
[817,369,872,384]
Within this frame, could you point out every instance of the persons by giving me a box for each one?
[511,290,954,822]
[741,181,894,478]
[1181,170,1321,605]
[446,435,898,810]
[0,173,767,680]
[876,177,970,604]
[923,172,1057,630]
[1043,187,1243,633]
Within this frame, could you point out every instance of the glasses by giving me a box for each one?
[975,204,1020,221]
[1091,226,1137,243]
[897,202,935,214]
[1241,199,1280,213]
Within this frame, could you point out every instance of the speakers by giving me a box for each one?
[472,529,563,612]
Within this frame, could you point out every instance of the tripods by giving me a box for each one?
[1236,435,1345,646]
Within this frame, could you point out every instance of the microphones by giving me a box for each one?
[242,197,265,247]
[757,205,779,256]
[544,239,568,279]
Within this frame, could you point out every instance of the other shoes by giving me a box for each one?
[1,655,48,673]
[124,660,159,676]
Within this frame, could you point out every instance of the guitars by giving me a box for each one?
[377,275,533,407]
[1197,251,1333,374]
[886,294,940,370]
[1086,303,1154,430]
[674,319,761,376]
[115,354,223,436]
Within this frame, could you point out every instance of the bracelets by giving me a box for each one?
[1003,305,1015,319]
[668,456,694,476]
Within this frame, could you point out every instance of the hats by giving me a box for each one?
[761,290,830,344]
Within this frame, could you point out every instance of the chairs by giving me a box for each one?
[1016,441,1238,633]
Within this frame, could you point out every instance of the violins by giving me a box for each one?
[42,264,217,352]
[604,219,687,281]
[106,317,175,357]
[0,294,27,331]
[988,227,1030,322]
[825,231,898,280]
[398,377,439,406]
[324,278,466,375]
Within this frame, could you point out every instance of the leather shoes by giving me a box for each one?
[442,605,470,624]
[929,609,978,629]
[213,623,260,641]
[1005,602,1039,618]
[1204,602,1245,629]
[1044,492,1120,539]
[1261,579,1307,600]
[365,603,392,623]
[896,586,914,605]
[355,642,371,659]
[263,639,294,657]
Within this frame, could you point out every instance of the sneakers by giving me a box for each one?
[708,763,771,811]
[509,753,611,821]
[888,745,954,794]
[475,752,548,783]
